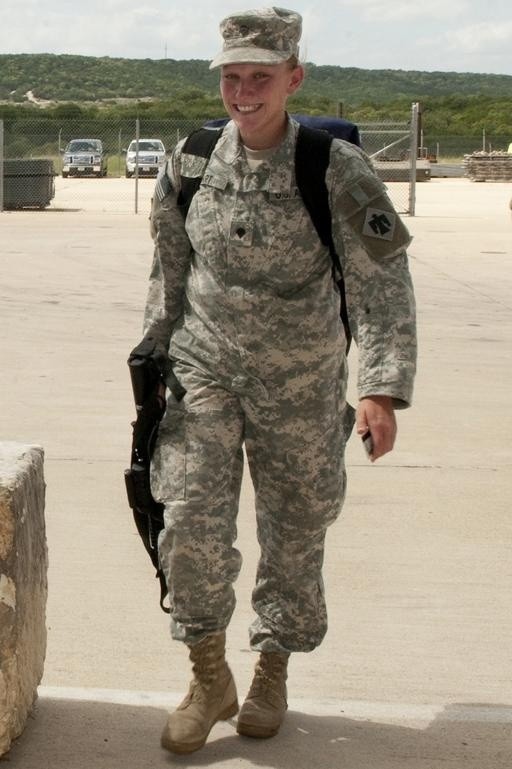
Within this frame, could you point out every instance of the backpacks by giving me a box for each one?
[178,115,360,355]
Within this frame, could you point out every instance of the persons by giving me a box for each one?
[122,5,419,758]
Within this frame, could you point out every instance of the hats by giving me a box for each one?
[208,7,302,70]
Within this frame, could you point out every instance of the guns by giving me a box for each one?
[126,333,173,593]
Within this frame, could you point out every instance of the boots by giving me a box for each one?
[237,653,290,738]
[161,632,238,755]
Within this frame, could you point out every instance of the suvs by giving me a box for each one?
[120,137,170,178]
[58,138,109,178]
